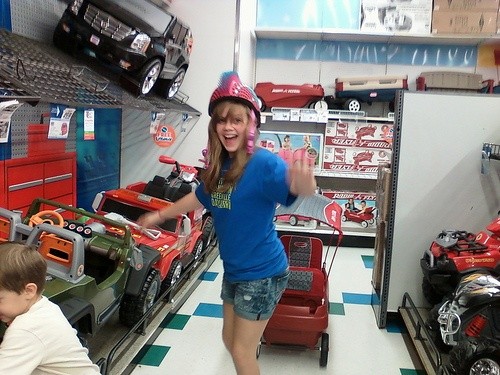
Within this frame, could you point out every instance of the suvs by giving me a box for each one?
[420,210,500,305]
[426,267,500,375]
[52,0,193,98]
[1,197,162,375]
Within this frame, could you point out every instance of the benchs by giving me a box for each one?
[283,265,326,298]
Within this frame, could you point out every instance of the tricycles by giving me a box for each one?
[257,194,343,368]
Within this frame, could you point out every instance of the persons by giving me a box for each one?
[136,71,317,375]
[0,241,102,375]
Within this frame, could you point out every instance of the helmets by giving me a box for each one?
[199,71,261,169]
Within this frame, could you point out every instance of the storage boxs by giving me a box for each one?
[255,0,500,39]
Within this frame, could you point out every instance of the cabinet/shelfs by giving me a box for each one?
[256,112,395,237]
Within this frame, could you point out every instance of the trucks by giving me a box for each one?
[85,156,215,304]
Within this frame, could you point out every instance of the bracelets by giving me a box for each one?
[158,210,166,222]
